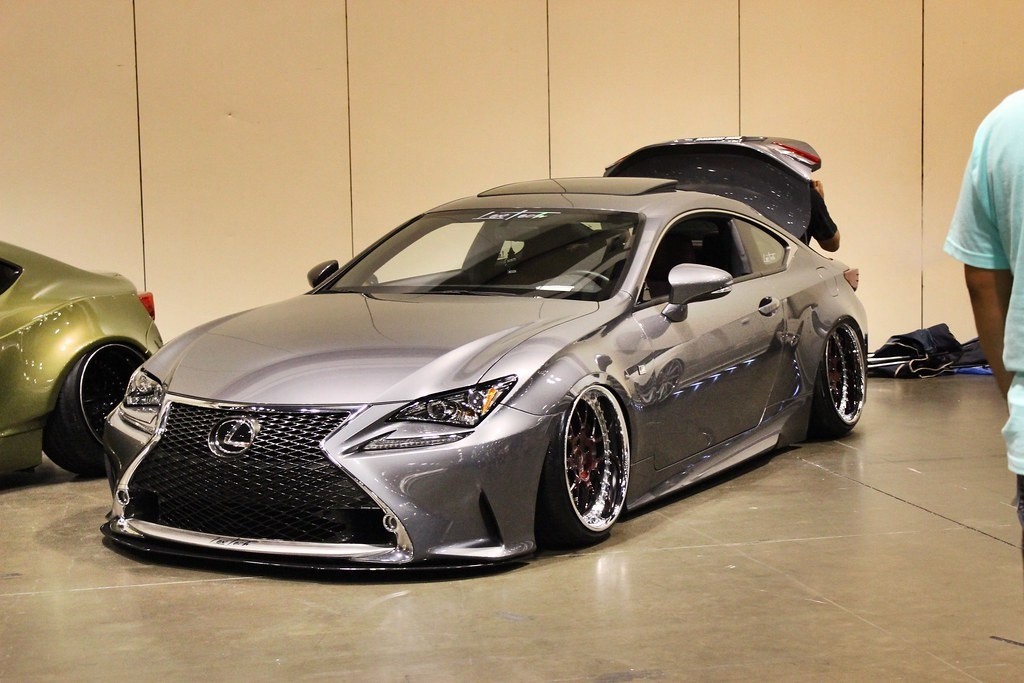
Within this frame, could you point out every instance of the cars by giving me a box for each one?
[0,242,162,486]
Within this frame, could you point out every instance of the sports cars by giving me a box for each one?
[101,134,870,571]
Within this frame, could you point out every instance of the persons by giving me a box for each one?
[798,180,841,252]
[942,88,1024,570]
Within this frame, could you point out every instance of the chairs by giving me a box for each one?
[648,228,725,282]
